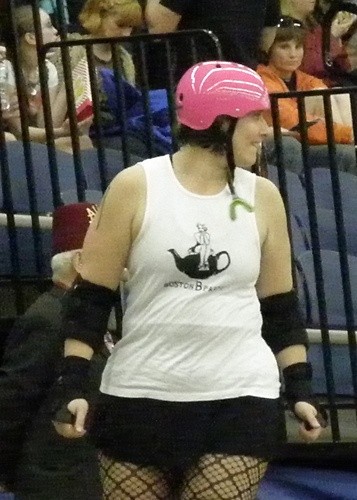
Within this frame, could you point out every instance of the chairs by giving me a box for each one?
[0,139,357,328]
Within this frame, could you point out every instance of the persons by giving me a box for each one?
[0,6,81,145]
[0,203,119,500]
[278,0,357,88]
[43,61,326,500]
[254,16,357,178]
[47,0,152,159]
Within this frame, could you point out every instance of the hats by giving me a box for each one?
[53,202,99,252]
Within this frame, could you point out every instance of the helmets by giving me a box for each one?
[175,61,270,131]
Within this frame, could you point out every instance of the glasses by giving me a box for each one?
[275,18,304,29]
